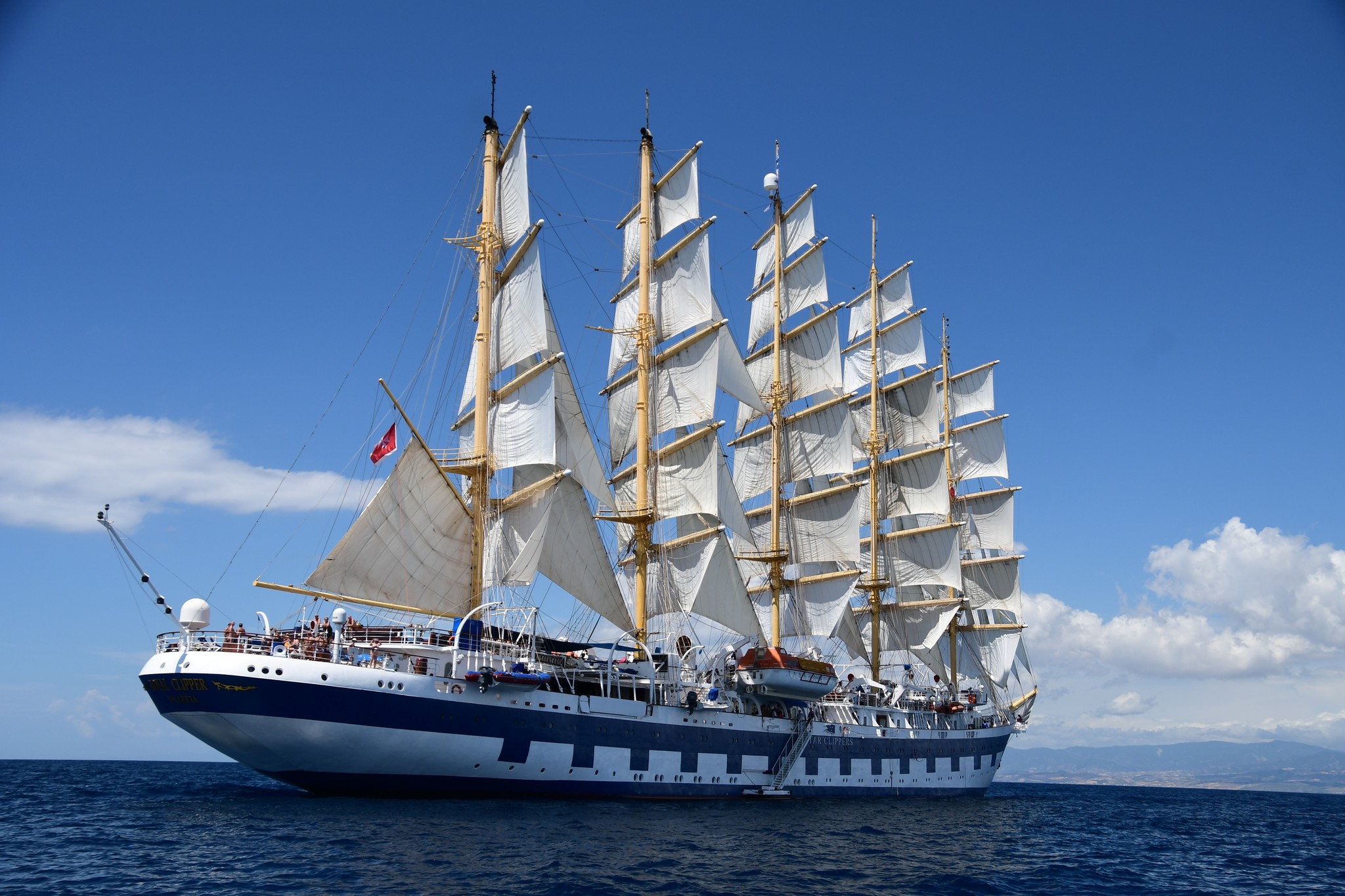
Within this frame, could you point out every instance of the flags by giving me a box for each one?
[950,480,958,498]
[370,422,398,466]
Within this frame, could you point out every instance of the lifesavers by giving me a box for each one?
[449,636,455,645]
[309,646,322,660]
[451,685,462,694]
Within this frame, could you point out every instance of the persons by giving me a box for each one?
[1017,714,1022,721]
[982,721,989,729]
[796,710,805,732]
[263,614,357,662]
[803,708,815,732]
[223,621,246,643]
[369,638,382,668]
[755,706,792,719]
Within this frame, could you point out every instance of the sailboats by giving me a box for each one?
[95,68,1038,804]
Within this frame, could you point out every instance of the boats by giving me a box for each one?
[735,646,838,699]
[464,671,552,693]
